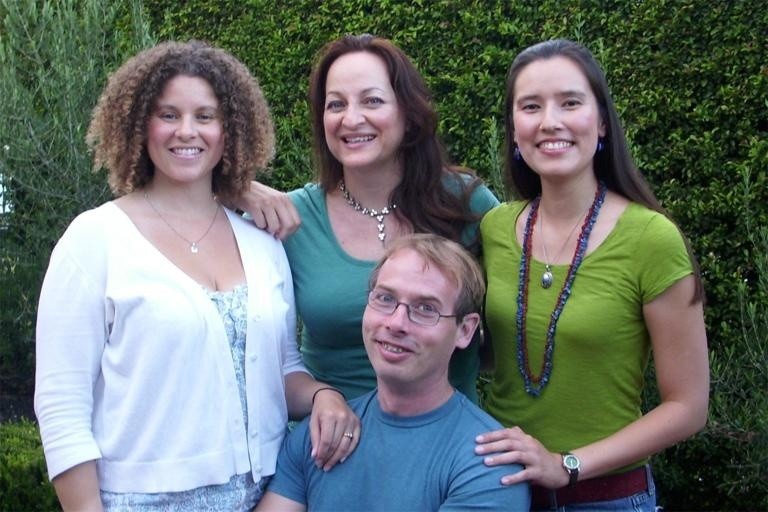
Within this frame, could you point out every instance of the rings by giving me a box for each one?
[343,433,353,438]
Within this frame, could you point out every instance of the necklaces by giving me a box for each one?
[538,204,590,287]
[515,183,608,398]
[338,178,396,241]
[141,184,220,252]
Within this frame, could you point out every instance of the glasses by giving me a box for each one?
[368,291,457,326]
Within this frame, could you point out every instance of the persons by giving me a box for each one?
[249,233,534,512]
[215,34,501,408]
[473,40,709,512]
[33,40,361,512]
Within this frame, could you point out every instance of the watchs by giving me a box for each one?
[559,450,581,487]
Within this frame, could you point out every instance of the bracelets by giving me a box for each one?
[311,387,347,405]
